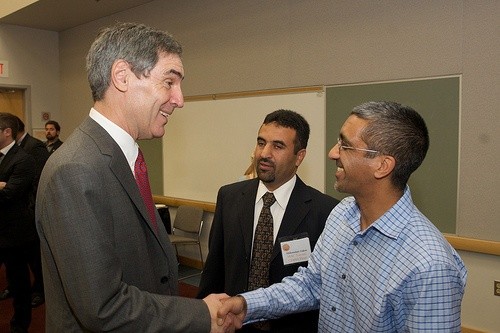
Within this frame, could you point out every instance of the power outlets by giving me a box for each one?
[494,281,500,296]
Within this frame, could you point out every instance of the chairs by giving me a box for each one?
[169,204,204,281]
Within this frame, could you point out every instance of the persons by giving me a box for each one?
[214,99,470,333]
[0,116,50,307]
[36,22,245,333]
[198,107,342,333]
[1,110,37,333]
[43,121,64,155]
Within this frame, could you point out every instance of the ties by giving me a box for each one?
[134,148,157,235]
[247,192,279,293]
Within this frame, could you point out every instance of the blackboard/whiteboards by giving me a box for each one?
[135,72,461,235]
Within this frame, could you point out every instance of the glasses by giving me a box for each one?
[337,138,383,155]
